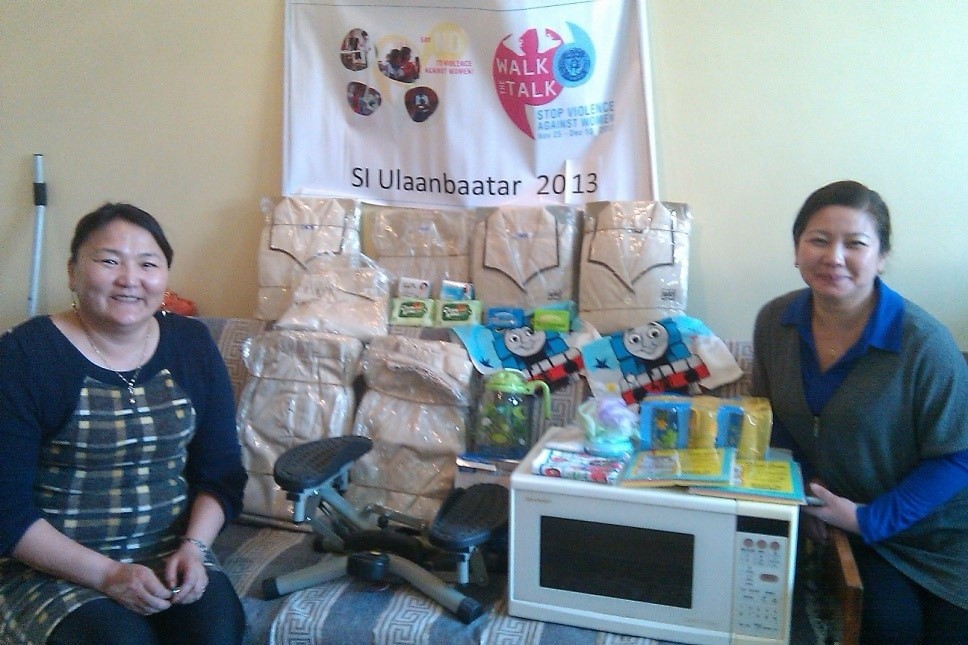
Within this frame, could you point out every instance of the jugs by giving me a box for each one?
[472,368,553,461]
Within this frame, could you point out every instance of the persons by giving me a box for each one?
[0,204,249,645]
[340,26,439,125]
[753,181,968,645]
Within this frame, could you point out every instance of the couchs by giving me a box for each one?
[165,312,863,645]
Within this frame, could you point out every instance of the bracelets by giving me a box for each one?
[181,536,209,563]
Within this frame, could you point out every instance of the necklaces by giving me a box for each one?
[74,307,153,404]
[814,308,863,358]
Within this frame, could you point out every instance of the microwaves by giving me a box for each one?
[506,425,804,645]
